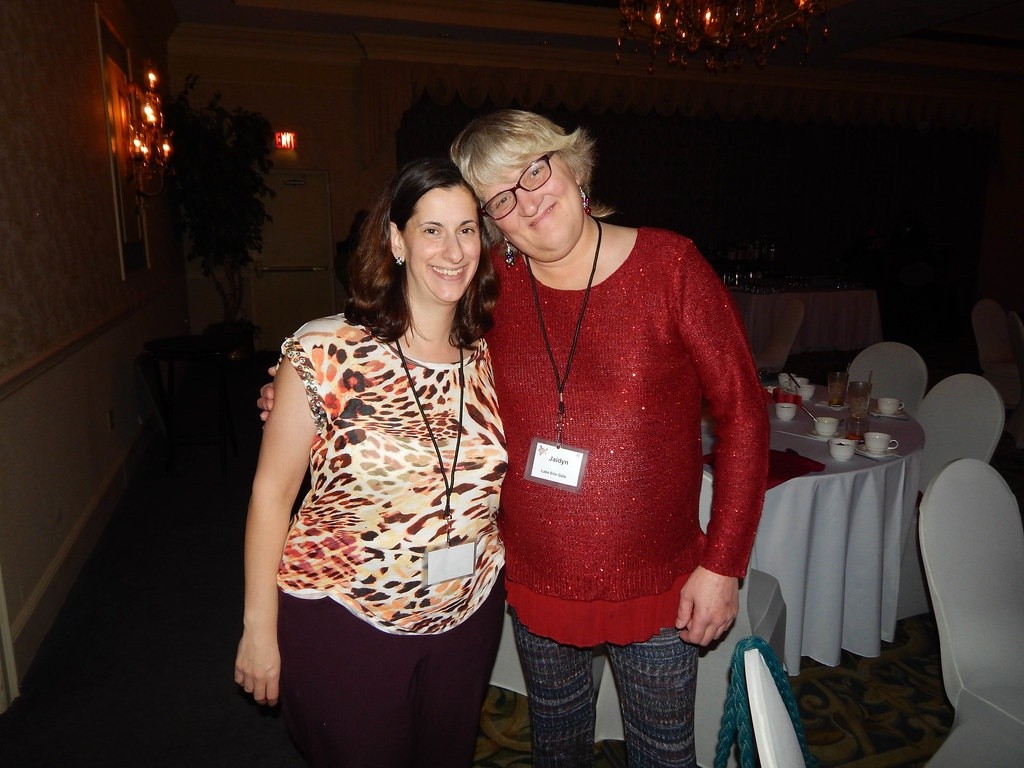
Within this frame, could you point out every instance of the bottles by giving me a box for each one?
[699,233,781,294]
[799,272,819,288]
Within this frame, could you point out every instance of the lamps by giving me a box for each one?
[614,0,831,75]
[120,58,176,215]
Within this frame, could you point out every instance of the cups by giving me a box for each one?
[878,398,904,414]
[849,381,872,419]
[847,418,870,442]
[828,372,848,406]
[864,432,898,452]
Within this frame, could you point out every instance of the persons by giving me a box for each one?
[236,160,508,768]
[256,110,770,768]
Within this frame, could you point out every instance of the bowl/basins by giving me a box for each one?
[828,438,857,461]
[815,417,839,435]
[778,373,796,386]
[775,403,796,422]
[790,378,809,391]
[796,385,815,400]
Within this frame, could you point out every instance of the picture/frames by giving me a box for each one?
[94,2,152,281]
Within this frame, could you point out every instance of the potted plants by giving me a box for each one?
[159,72,275,372]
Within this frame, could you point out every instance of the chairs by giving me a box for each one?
[745,297,1024,767]
[136,353,230,482]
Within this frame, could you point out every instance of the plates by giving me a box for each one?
[856,446,898,457]
[807,430,847,439]
[870,412,904,417]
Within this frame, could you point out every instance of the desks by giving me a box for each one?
[144,335,238,463]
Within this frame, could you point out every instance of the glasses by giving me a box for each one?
[479,151,556,220]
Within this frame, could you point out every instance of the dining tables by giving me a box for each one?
[698,379,931,675]
[728,277,884,361]
